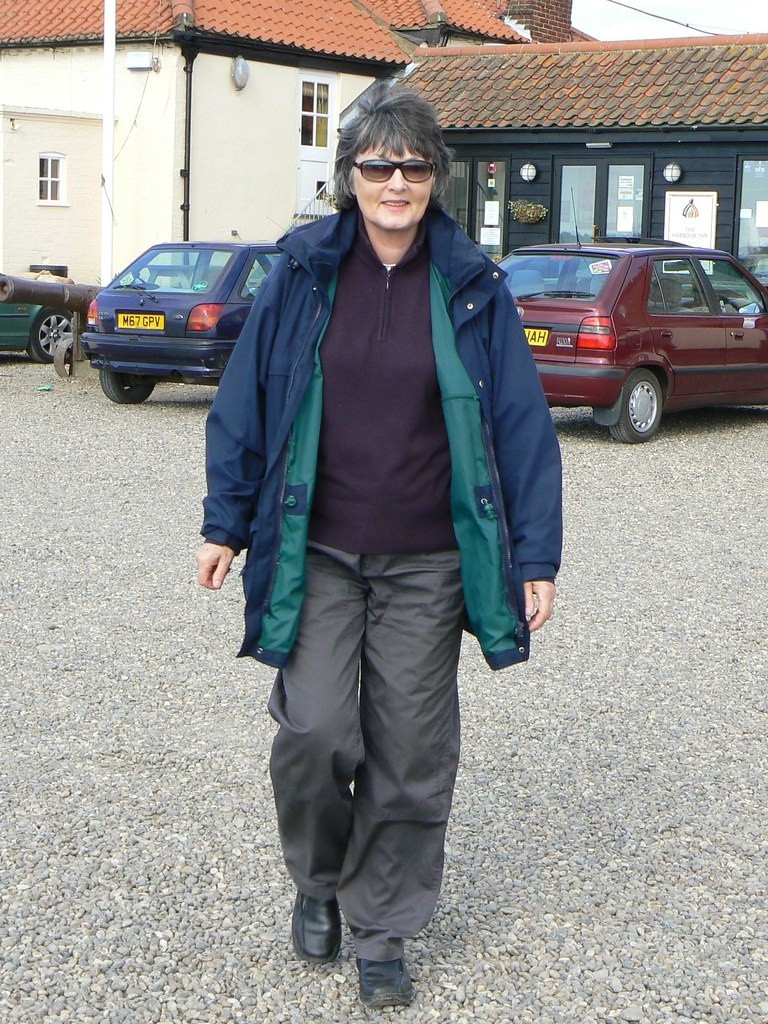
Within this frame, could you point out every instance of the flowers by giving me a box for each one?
[508,199,548,220]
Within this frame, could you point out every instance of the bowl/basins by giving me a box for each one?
[625,238,641,243]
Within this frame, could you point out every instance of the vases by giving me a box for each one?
[518,210,541,224]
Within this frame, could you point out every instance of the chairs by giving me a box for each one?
[589,274,608,296]
[648,278,692,314]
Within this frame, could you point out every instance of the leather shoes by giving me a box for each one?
[292,890,342,963]
[356,956,412,1007]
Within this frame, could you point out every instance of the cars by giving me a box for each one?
[79,240,286,407]
[483,234,768,444]
[0,273,75,365]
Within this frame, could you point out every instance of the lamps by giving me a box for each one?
[519,162,538,183]
[231,56,249,92]
[662,161,683,184]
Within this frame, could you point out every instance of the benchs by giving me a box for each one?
[507,270,546,298]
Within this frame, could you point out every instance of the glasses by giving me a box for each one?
[353,159,435,183]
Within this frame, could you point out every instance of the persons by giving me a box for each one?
[196,97,565,1001]
